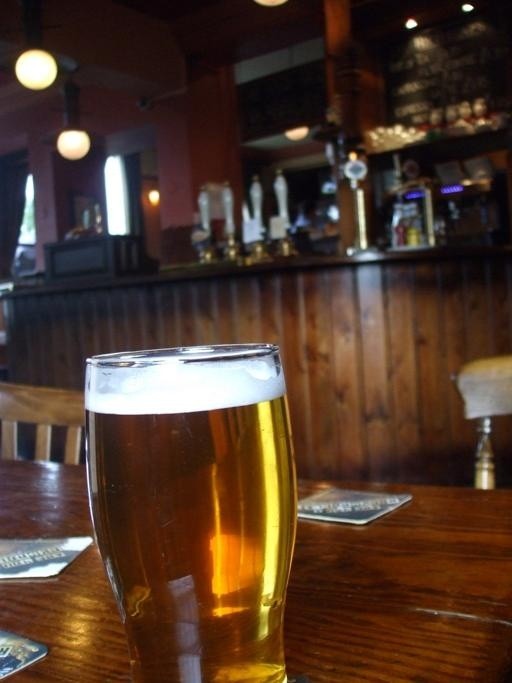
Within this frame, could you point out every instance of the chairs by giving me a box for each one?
[446,355,512,490]
[0,373,86,466]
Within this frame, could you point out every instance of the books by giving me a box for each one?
[0,629,49,679]
[292,487,414,526]
[0,535,94,579]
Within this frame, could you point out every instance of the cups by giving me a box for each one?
[84,342,297,682]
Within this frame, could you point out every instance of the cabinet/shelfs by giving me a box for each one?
[241,130,512,246]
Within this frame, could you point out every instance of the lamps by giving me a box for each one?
[52,74,99,166]
[13,0,60,92]
